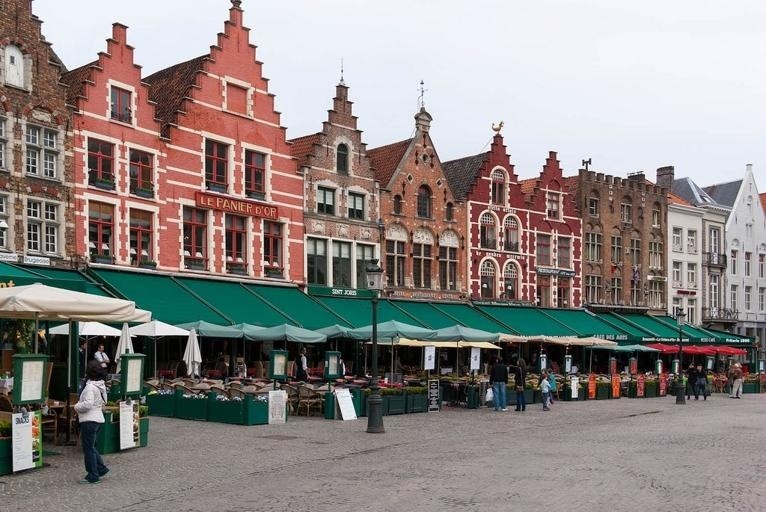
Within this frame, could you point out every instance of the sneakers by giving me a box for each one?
[79,478,101,484]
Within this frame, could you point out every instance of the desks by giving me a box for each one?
[309,388,329,417]
[190,386,213,392]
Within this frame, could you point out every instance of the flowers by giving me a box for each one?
[180,392,209,401]
[216,394,241,403]
[148,388,172,395]
[252,394,268,403]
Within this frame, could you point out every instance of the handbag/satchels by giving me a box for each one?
[517,386,523,392]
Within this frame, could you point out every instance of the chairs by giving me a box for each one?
[141,358,650,419]
[0,392,85,461]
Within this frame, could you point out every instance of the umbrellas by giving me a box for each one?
[134,307,153,323]
[181,328,203,378]
[0,284,134,443]
[113,323,135,374]
[37,320,123,387]
[126,318,190,381]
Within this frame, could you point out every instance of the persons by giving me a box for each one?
[694,363,709,401]
[729,363,744,399]
[685,362,697,400]
[514,358,527,412]
[439,356,448,369]
[78,342,88,377]
[74,359,110,485]
[295,346,311,384]
[94,343,110,372]
[337,357,346,380]
[538,367,553,405]
[394,355,402,372]
[540,374,552,411]
[490,355,511,411]
[547,368,557,404]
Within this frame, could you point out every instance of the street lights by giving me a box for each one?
[364,257,387,433]
[674,308,688,406]
